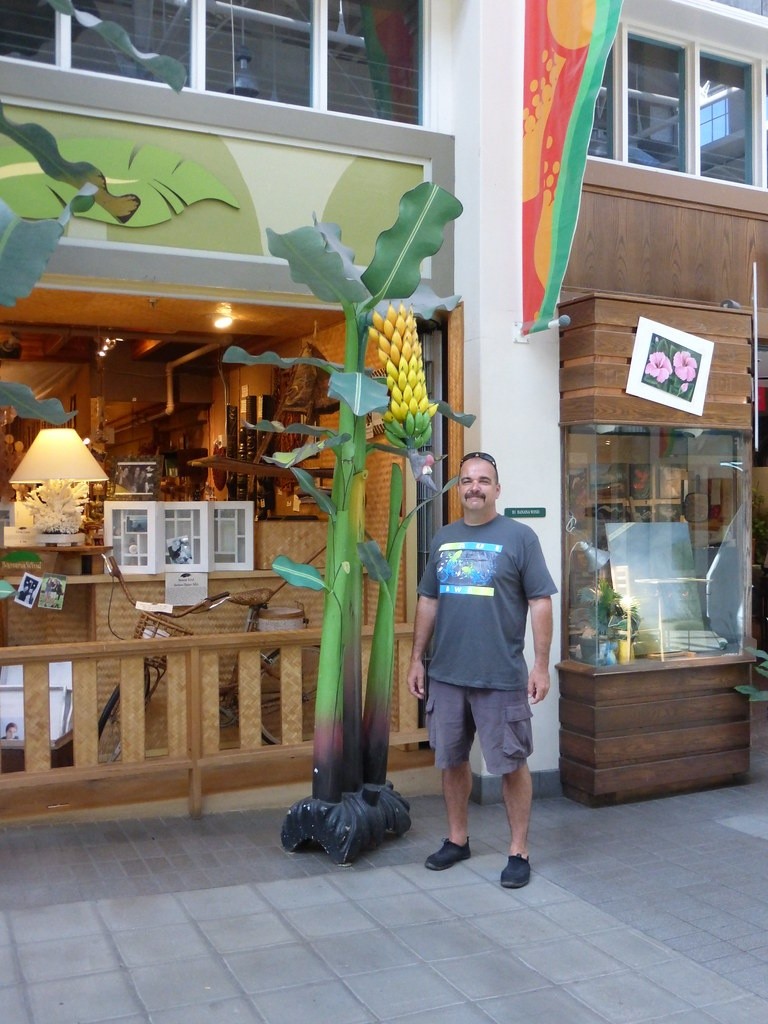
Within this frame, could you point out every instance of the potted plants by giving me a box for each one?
[572,579,642,665]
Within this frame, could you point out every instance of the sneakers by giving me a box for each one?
[500,853,531,887]
[425,836,471,871]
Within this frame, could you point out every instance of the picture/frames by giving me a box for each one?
[70,394,76,428]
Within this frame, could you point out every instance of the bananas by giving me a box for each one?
[366,300,440,450]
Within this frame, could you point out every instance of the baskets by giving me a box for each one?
[132,611,193,669]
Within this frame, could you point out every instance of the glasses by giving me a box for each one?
[460,452,496,470]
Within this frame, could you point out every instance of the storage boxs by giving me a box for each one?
[114,461,156,495]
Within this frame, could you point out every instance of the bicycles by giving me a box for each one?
[98,555,322,762]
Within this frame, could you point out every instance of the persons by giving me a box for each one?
[1,722,18,740]
[17,573,64,609]
[116,466,152,494]
[169,539,193,564]
[406,451,558,889]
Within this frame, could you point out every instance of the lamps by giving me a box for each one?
[569,541,611,573]
[9,428,109,547]
[227,0,260,98]
